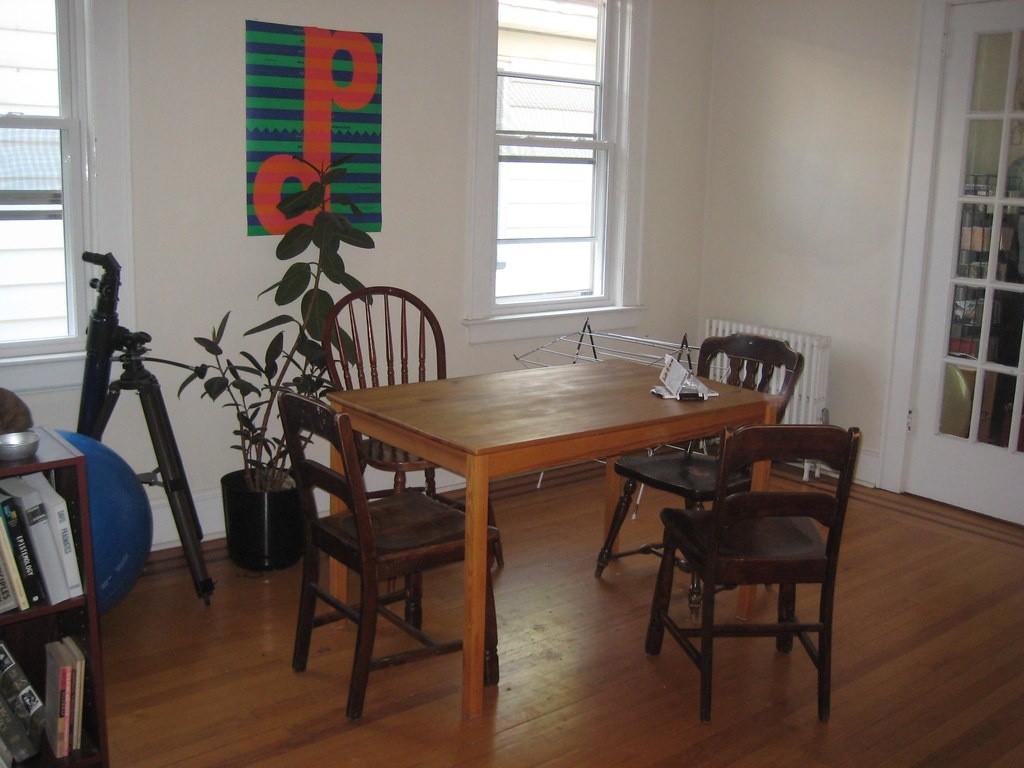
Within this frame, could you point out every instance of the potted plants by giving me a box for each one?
[160,147,376,571]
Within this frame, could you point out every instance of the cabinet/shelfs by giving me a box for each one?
[0,427,109,768]
[947,175,1021,442]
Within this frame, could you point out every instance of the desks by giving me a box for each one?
[326,361,786,719]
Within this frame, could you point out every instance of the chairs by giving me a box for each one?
[277,287,861,722]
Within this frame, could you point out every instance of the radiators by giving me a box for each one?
[704,321,832,481]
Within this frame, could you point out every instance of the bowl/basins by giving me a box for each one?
[0,431,42,462]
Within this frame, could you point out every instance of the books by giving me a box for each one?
[0,642,46,768]
[0,472,85,613]
[45,636,87,758]
